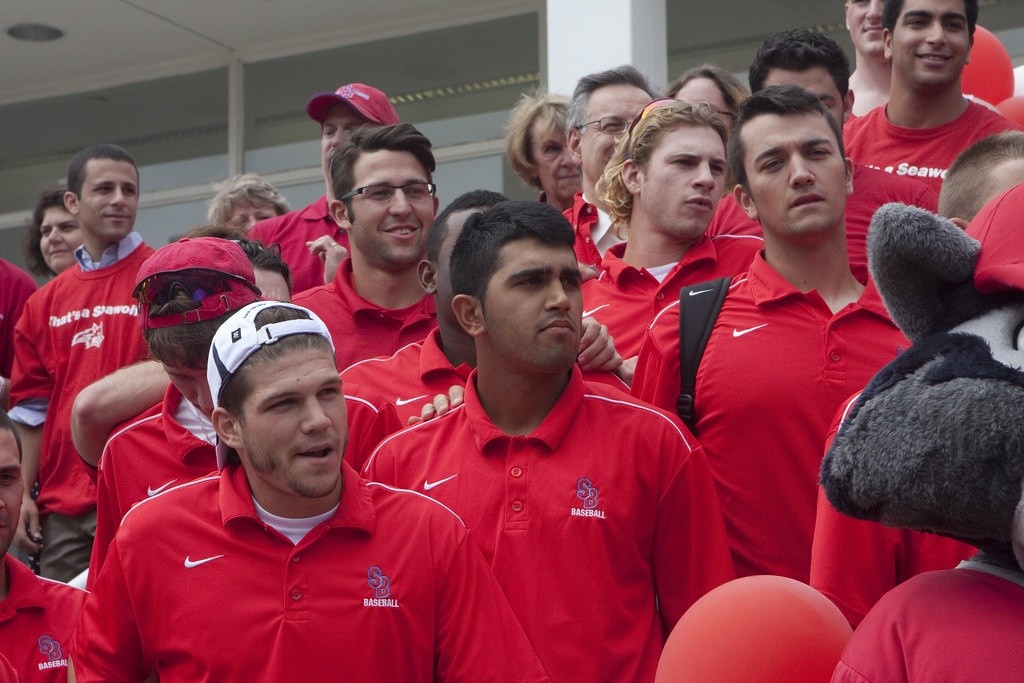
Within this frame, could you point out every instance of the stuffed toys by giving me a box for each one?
[819,182,1024,683]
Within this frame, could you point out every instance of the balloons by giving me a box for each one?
[996,95,1024,127]
[655,574,853,683]
[961,23,1015,107]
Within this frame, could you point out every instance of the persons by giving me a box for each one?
[0,0,1024,592]
[582,97,765,390]
[357,201,736,682]
[86,236,465,595]
[629,84,913,584]
[0,405,92,683]
[68,299,549,683]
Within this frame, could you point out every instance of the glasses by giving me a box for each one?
[340,183,436,203]
[575,116,632,135]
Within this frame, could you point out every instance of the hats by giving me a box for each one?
[132,237,261,332]
[206,300,335,471]
[306,83,399,128]
